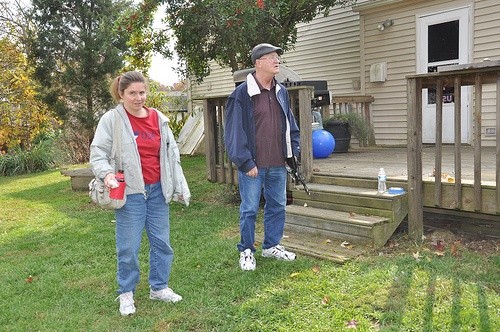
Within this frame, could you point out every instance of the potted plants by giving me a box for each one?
[323,112,375,154]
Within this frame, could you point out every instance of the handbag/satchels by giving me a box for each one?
[88,177,127,210]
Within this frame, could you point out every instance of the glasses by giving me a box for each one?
[259,56,279,62]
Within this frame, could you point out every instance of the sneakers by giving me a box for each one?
[262,244,297,261]
[149,285,182,303]
[115,290,136,316]
[239,248,256,271]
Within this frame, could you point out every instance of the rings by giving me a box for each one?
[246,173,248,175]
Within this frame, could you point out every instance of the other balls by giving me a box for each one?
[312,129,336,158]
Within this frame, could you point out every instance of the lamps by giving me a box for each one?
[376,19,393,31]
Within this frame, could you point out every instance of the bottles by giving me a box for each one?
[377,168,387,194]
[108,169,126,199]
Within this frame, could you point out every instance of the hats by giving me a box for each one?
[250,43,284,64]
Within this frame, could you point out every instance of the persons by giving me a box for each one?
[90,71,192,315]
[223,43,300,271]
